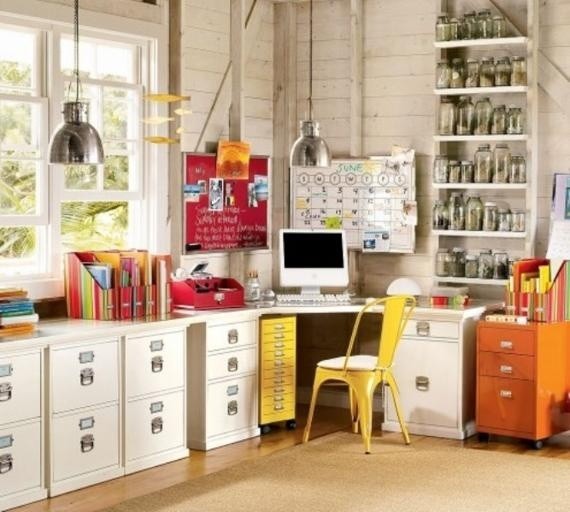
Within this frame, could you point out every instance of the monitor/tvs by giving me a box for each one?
[278,227,349,295]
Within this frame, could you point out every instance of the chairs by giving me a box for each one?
[301,296,416,454]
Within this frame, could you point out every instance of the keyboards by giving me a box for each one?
[276,293,354,310]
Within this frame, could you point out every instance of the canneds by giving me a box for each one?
[497,208,512,232]
[493,15,506,37]
[506,257,520,274]
[508,155,526,183]
[483,202,499,231]
[432,156,449,184]
[465,254,479,278]
[460,159,473,184]
[448,160,461,181]
[511,208,526,234]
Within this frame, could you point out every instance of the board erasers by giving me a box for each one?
[186,243,202,251]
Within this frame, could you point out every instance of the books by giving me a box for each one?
[509,259,552,294]
[0,288,39,342]
[83,252,152,290]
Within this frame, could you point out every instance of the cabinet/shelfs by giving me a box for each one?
[259,313,296,425]
[127,329,186,467]
[0,344,46,503]
[477,318,570,450]
[430,0,537,288]
[188,310,260,452]
[49,338,122,494]
[385,314,462,439]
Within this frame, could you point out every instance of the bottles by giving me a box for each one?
[432,9,526,279]
[244,278,260,301]
[261,289,275,306]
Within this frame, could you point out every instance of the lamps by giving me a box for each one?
[289,0,330,168]
[49,2,104,164]
